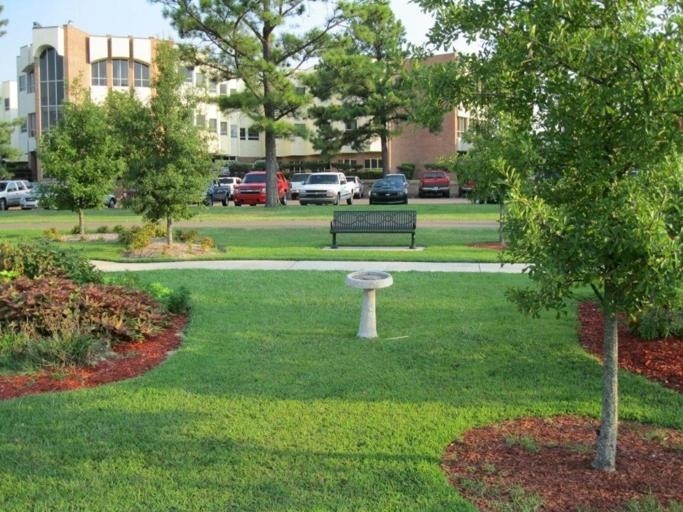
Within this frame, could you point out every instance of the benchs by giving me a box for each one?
[330,210,417,249]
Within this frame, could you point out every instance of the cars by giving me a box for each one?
[368,173,409,204]
[419,169,449,198]
[20,185,39,209]
[460,180,474,195]
[208,171,364,206]
[105,195,117,208]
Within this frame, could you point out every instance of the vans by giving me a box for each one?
[0,179,32,210]
